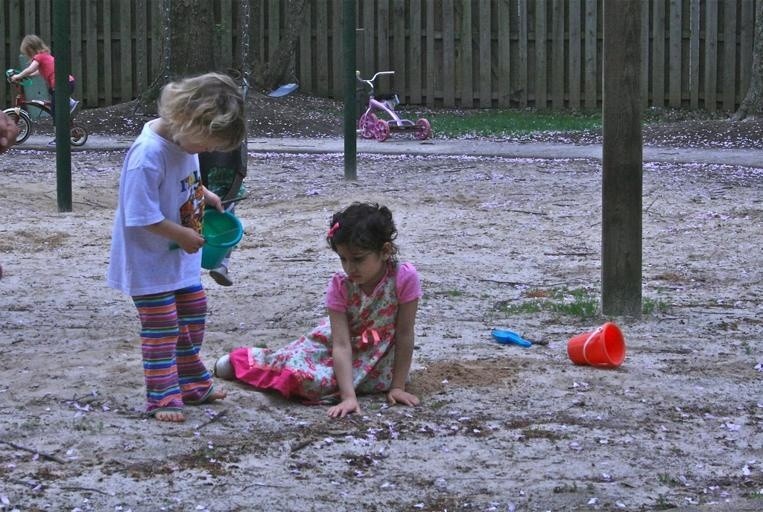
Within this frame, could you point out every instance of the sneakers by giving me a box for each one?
[48,140,56,145]
[213,354,233,377]
[210,266,233,287]
[70,100,81,118]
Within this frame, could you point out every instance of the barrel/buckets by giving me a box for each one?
[201,208,243,269]
[568,321,625,365]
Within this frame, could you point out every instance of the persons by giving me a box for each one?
[198,140,247,286]
[0,110,18,153]
[214,202,421,420]
[104,72,246,424]
[12,34,81,147]
